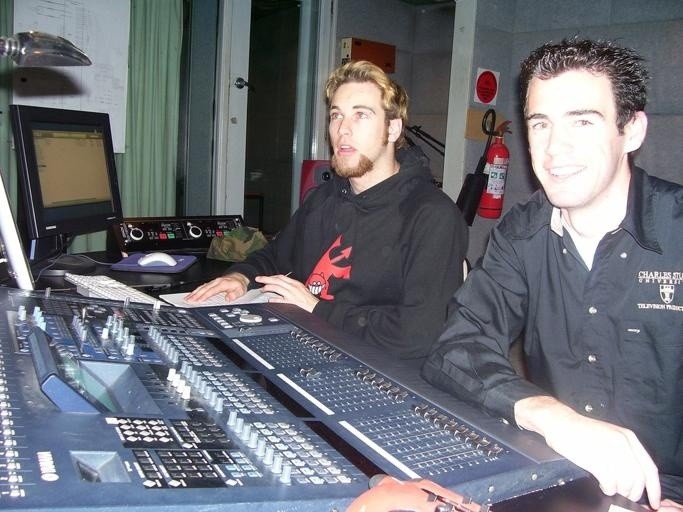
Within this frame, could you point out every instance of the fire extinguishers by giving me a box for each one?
[477,110,514,218]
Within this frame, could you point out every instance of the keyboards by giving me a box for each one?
[64,272,171,306]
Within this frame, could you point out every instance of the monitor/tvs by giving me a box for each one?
[8,104,125,276]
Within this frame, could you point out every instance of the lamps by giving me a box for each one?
[0,31,93,292]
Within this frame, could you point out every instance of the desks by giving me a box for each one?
[77,250,654,512]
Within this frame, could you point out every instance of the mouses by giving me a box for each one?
[138,252,177,268]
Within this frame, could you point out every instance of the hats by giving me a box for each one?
[207,227,268,263]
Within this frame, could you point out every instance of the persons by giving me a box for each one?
[421,35,683,510]
[184,59,469,370]
